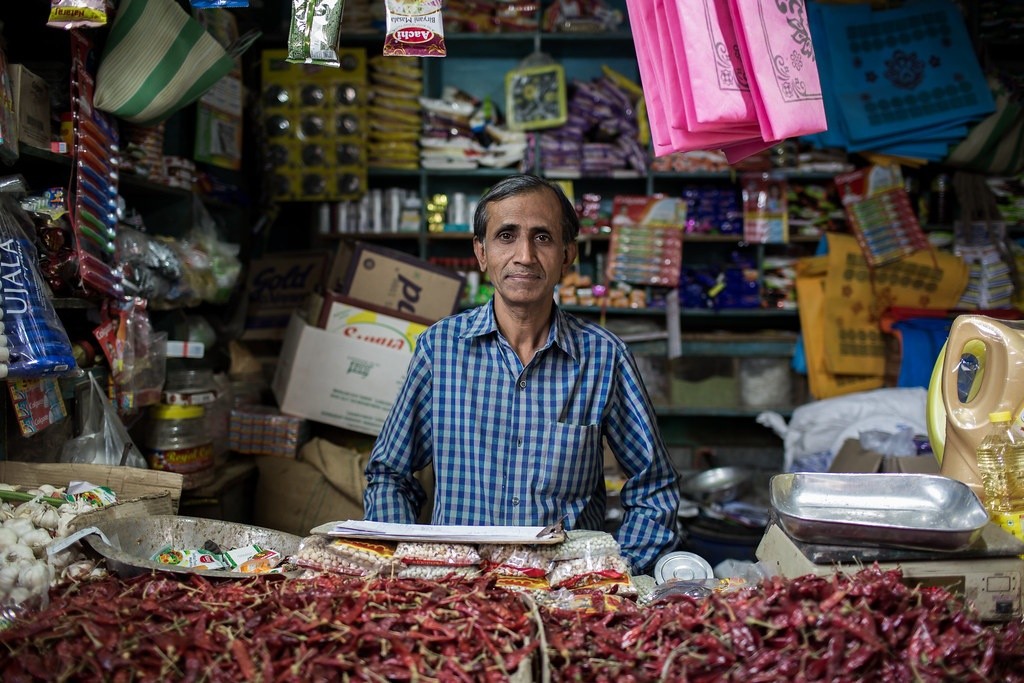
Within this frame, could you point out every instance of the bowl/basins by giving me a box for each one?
[681,466,755,503]
[83,515,305,577]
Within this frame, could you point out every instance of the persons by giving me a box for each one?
[362,175,682,577]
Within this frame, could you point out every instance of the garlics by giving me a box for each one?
[0,483,109,607]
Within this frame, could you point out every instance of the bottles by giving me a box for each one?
[976,411,1024,561]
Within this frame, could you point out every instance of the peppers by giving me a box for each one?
[0,559,1024,683]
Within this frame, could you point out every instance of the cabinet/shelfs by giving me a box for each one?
[262,27,856,500]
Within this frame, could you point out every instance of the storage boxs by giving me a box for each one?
[827,437,941,479]
[159,72,244,171]
[227,404,302,460]
[5,62,52,149]
[269,237,465,438]
[0,459,184,537]
